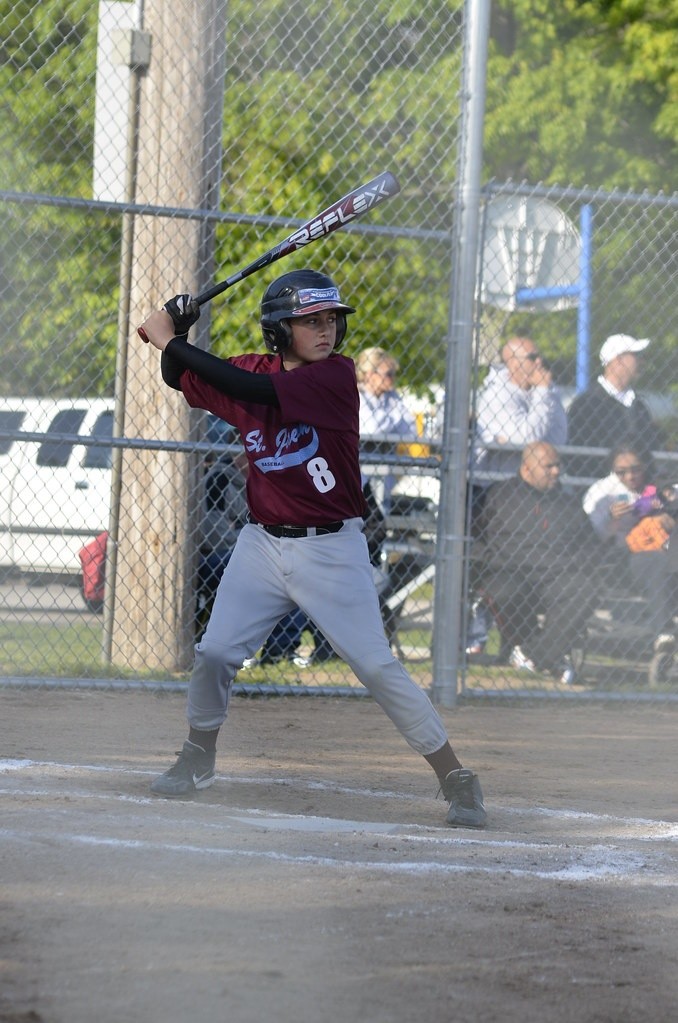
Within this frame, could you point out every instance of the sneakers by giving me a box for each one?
[150,739,216,794]
[436,768,488,829]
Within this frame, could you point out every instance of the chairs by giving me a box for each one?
[379,566,440,659]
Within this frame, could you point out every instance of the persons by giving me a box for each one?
[355,348,417,565]
[142,270,488,829]
[568,334,668,515]
[582,442,678,685]
[464,339,567,656]
[476,442,595,685]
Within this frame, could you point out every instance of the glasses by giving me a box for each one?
[507,353,540,363]
[614,463,647,478]
[376,369,401,378]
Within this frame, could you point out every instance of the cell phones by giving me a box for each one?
[618,494,628,502]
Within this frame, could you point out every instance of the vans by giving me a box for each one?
[2,395,118,578]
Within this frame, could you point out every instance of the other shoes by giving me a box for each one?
[653,633,677,654]
[465,643,483,657]
[509,645,538,674]
[549,662,575,683]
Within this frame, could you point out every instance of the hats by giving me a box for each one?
[600,334,650,366]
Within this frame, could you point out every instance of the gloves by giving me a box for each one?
[159,294,200,336]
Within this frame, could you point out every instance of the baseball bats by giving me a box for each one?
[136,172,403,345]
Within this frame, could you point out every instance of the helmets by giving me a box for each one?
[261,269,356,354]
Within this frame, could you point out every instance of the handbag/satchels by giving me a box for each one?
[624,512,677,553]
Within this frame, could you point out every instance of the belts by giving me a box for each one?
[248,516,343,538]
